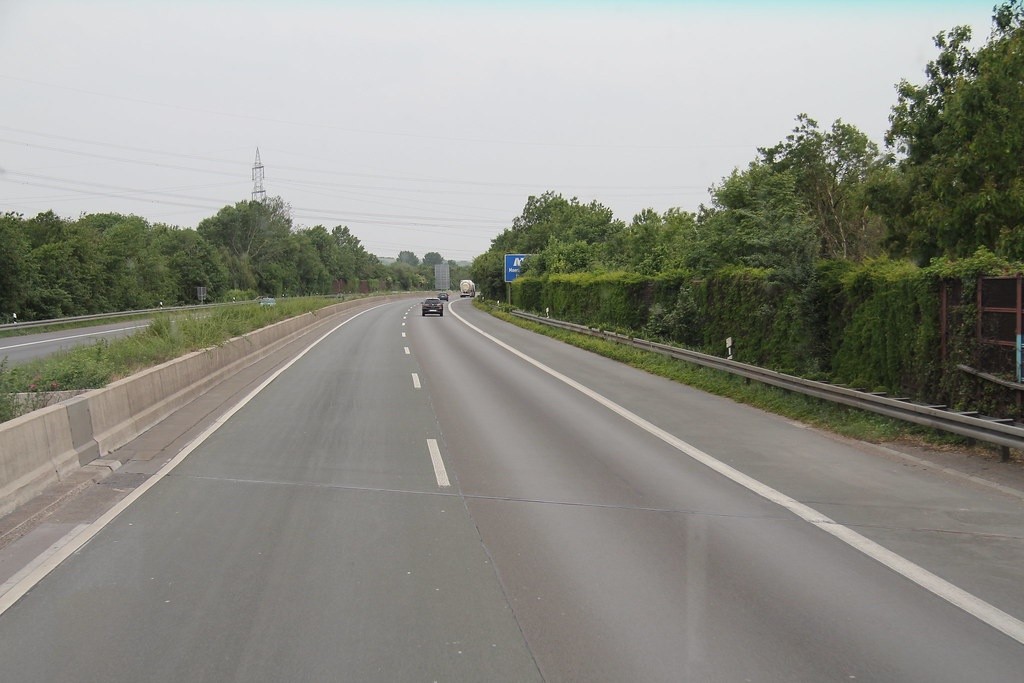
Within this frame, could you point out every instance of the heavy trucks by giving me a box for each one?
[459,279,476,297]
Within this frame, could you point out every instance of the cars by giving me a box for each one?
[438,293,448,301]
[421,298,444,316]
